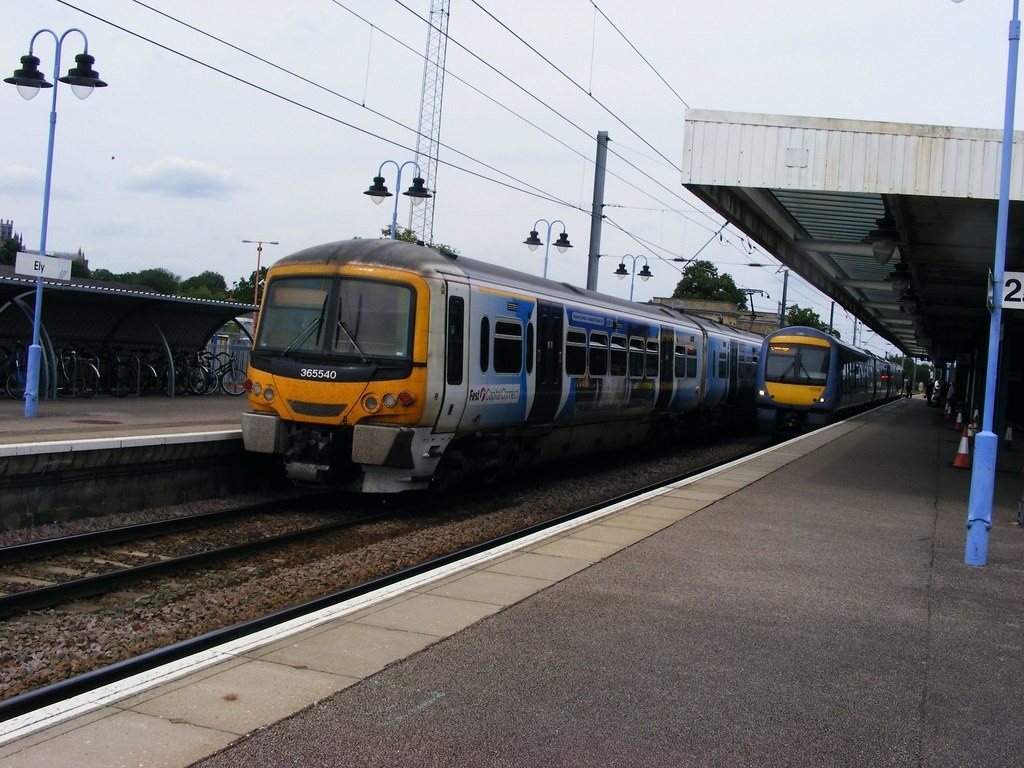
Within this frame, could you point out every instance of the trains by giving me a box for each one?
[242,238,764,508]
[757,326,904,430]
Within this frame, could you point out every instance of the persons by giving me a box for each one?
[906,379,912,398]
[925,378,956,416]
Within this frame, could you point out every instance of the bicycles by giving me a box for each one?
[6,338,248,398]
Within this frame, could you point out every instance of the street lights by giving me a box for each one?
[612,254,654,304]
[241,239,279,339]
[2,27,109,417]
[523,219,572,280]
[364,159,433,239]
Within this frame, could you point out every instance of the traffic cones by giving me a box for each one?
[941,401,951,416]
[946,405,953,420]
[967,418,975,447]
[1002,423,1015,449]
[951,410,964,432]
[972,414,983,433]
[950,425,972,470]
